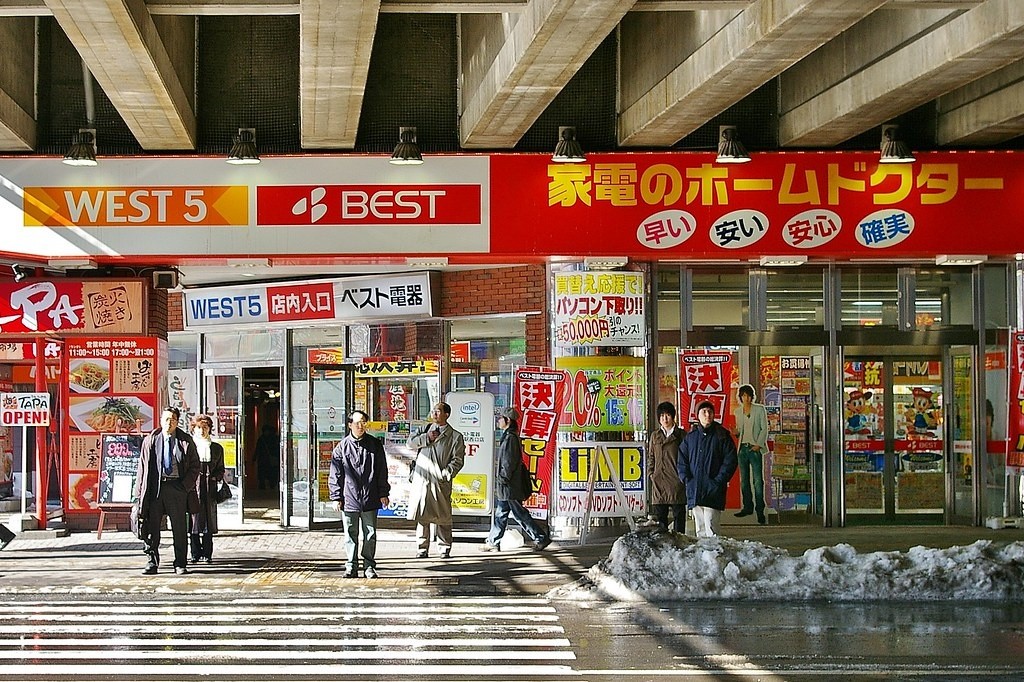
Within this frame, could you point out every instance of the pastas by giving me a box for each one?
[71,362,109,390]
[85,413,121,430]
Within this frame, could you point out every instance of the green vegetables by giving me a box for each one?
[91,396,142,425]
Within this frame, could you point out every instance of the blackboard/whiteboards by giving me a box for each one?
[97,432,150,507]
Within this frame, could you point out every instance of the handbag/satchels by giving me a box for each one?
[409,460,416,483]
[216,479,232,504]
[130,499,144,539]
[521,462,533,501]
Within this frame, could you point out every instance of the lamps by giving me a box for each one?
[389,131,424,165]
[11,263,34,277]
[879,129,917,163]
[225,130,261,164]
[550,129,587,162]
[715,129,752,163]
[62,132,98,167]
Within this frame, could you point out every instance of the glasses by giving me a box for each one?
[350,421,367,427]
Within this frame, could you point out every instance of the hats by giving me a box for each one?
[497,406,520,420]
[695,401,714,418]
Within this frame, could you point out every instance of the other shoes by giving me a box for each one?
[364,567,377,578]
[191,554,201,562]
[142,565,158,574]
[733,511,751,518]
[416,552,429,558]
[757,513,766,524]
[175,566,188,574]
[531,538,552,551]
[478,543,500,552]
[342,569,359,578]
[440,553,449,559]
[203,557,212,562]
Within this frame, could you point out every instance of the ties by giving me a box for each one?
[436,427,440,431]
[163,436,173,476]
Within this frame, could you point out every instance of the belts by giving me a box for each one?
[162,477,180,481]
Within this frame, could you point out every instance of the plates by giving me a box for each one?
[69,396,154,432]
[69,359,110,393]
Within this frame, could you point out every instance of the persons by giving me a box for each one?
[253,422,280,489]
[985,399,1003,482]
[678,401,737,536]
[329,410,390,579]
[479,406,553,553]
[405,401,465,558]
[131,407,200,575]
[736,384,769,524]
[649,402,687,534]
[189,414,225,562]
